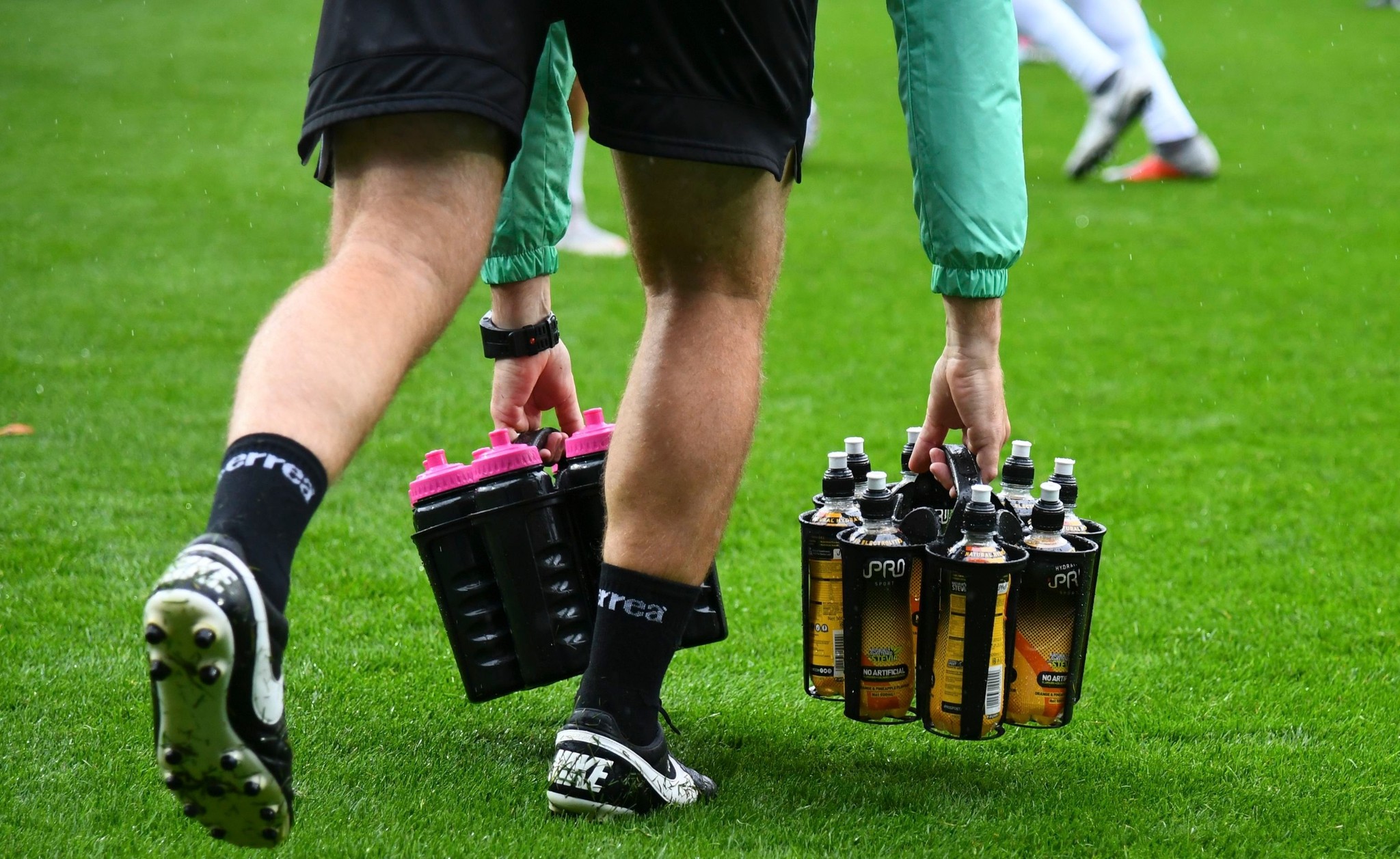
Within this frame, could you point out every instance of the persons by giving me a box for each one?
[1012,0,1221,183]
[555,72,819,258]
[143,0,1028,849]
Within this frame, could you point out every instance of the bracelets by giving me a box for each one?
[479,309,560,359]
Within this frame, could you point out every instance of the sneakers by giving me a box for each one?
[546,706,718,815]
[141,533,295,849]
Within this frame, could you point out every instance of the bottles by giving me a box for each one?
[406,406,721,699]
[891,426,924,495]
[1025,457,1087,533]
[807,451,864,699]
[996,439,1036,527]
[845,471,916,721]
[844,436,872,500]
[928,484,1011,736]
[1006,480,1077,725]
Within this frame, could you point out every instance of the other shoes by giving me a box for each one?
[1066,68,1152,183]
[1100,136,1219,182]
